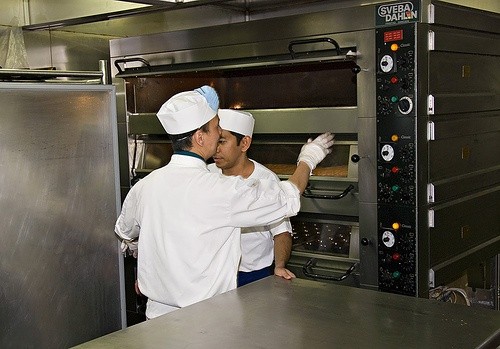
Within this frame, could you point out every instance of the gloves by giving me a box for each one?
[297,131,335,172]
[120,240,138,259]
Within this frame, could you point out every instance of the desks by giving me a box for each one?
[68,275,500,349]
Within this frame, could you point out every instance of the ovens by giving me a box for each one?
[109,0,499,309]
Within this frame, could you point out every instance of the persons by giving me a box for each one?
[114,85,335,321]
[207,109,297,288]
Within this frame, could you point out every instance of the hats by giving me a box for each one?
[156,85,219,135]
[217,108,255,138]
